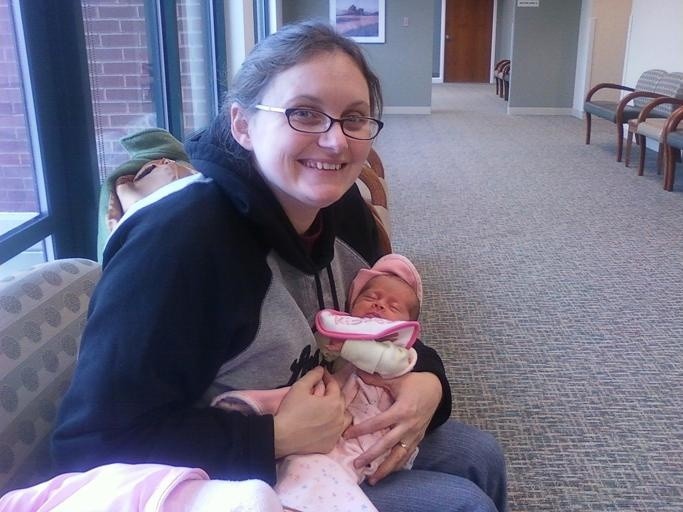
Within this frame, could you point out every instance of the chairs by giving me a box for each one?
[1,256,100,486]
[583,68,683,191]
[494,59,512,101]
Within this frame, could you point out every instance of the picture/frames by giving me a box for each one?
[329,0,385,44]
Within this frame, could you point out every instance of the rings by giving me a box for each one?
[398,442,410,452]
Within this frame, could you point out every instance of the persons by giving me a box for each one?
[208,253,423,489]
[48,17,508,511]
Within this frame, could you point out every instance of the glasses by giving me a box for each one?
[253,103,386,143]
